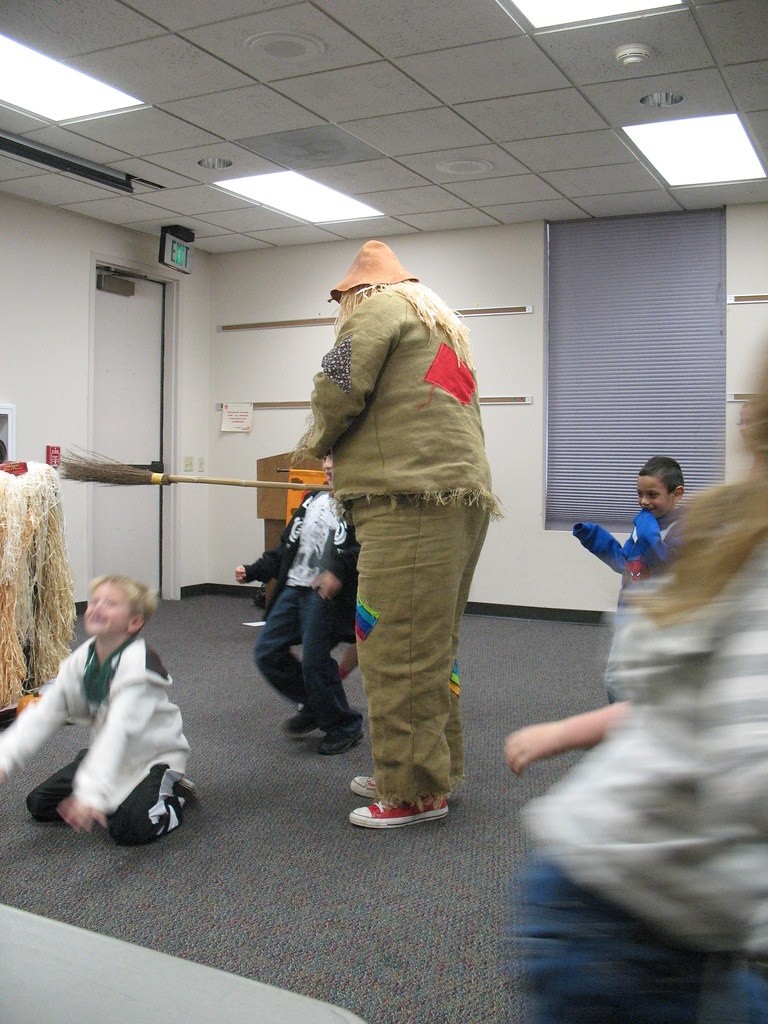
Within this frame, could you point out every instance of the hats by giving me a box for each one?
[330,240,420,305]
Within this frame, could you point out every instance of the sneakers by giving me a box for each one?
[350,776,378,798]
[350,792,448,828]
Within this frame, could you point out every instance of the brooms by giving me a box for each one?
[57,442,334,491]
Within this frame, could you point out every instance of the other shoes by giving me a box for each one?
[173,778,200,805]
[289,707,321,735]
[316,727,363,755]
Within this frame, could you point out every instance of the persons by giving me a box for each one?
[235,452,364,755]
[0,575,199,847]
[504,346,768,1024]
[298,240,503,828]
